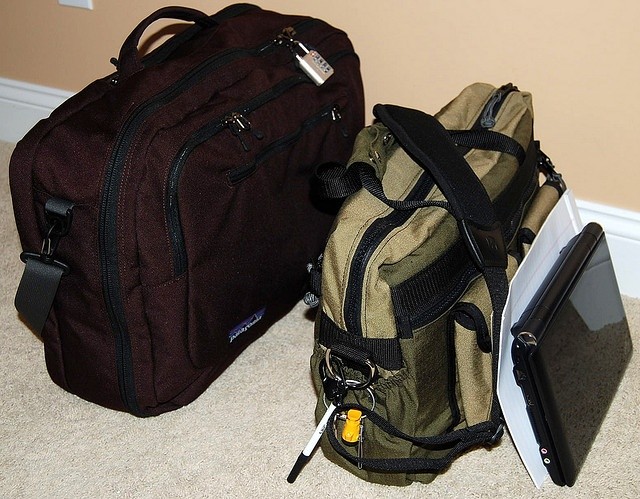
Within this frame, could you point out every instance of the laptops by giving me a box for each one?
[510,222,633,488]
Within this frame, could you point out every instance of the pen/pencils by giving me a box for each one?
[287,395,340,484]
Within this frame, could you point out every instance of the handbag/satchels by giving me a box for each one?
[9,3,364,418]
[287,83,567,487]
